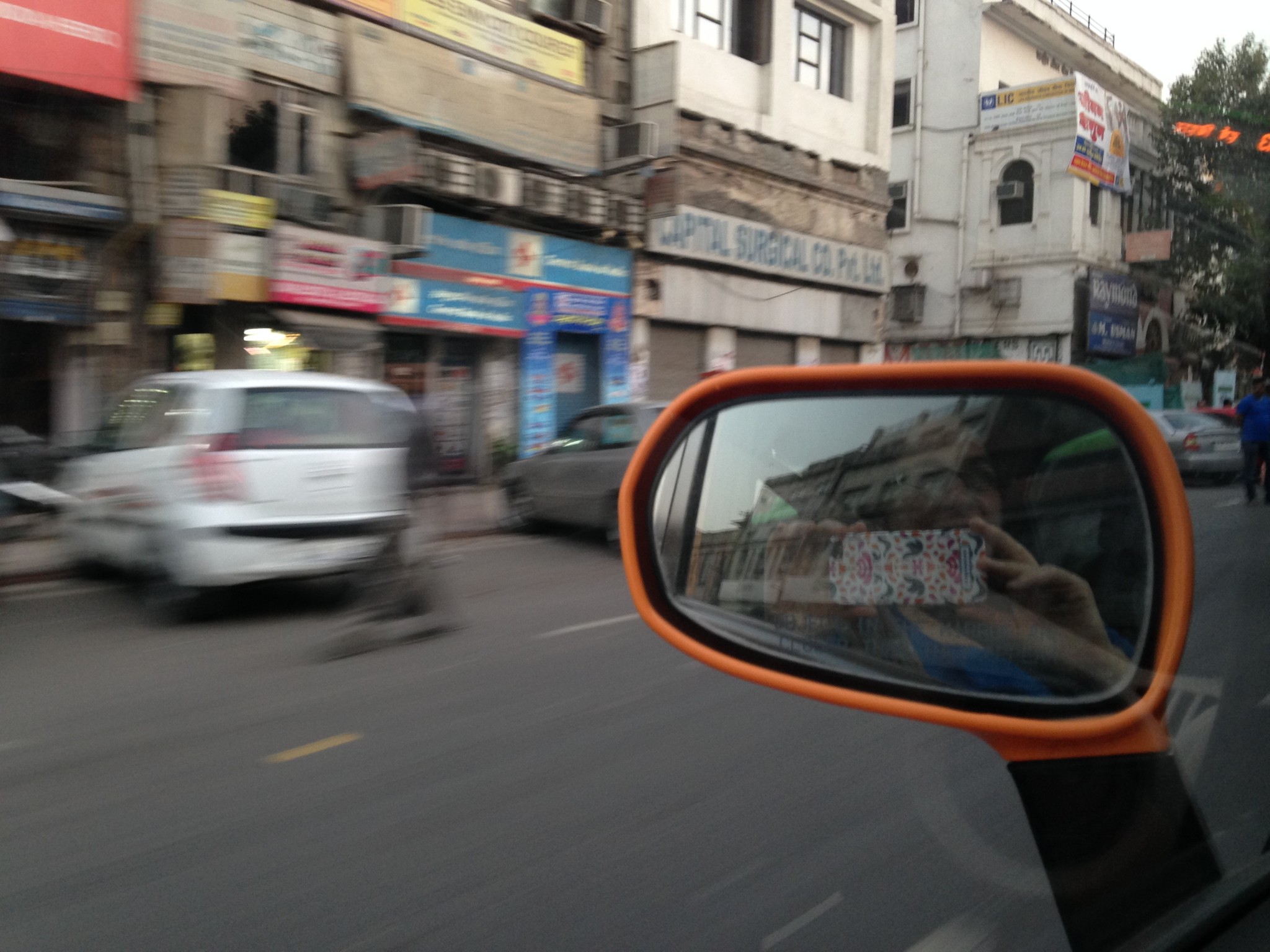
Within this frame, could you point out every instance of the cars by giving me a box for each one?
[38,370,431,617]
[1147,410,1245,482]
[1197,408,1241,428]
[504,402,674,552]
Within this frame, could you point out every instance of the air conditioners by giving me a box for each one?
[996,181,1026,198]
[521,169,569,219]
[603,120,661,161]
[364,202,435,253]
[888,181,908,200]
[415,144,476,195]
[292,188,342,230]
[604,193,648,235]
[472,159,525,208]
[565,182,610,228]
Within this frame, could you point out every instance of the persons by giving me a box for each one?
[1235,374,1270,507]
[760,398,1147,710]
[1221,399,1233,410]
[1197,400,1207,410]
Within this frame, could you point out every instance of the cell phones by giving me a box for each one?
[827,531,988,611]
